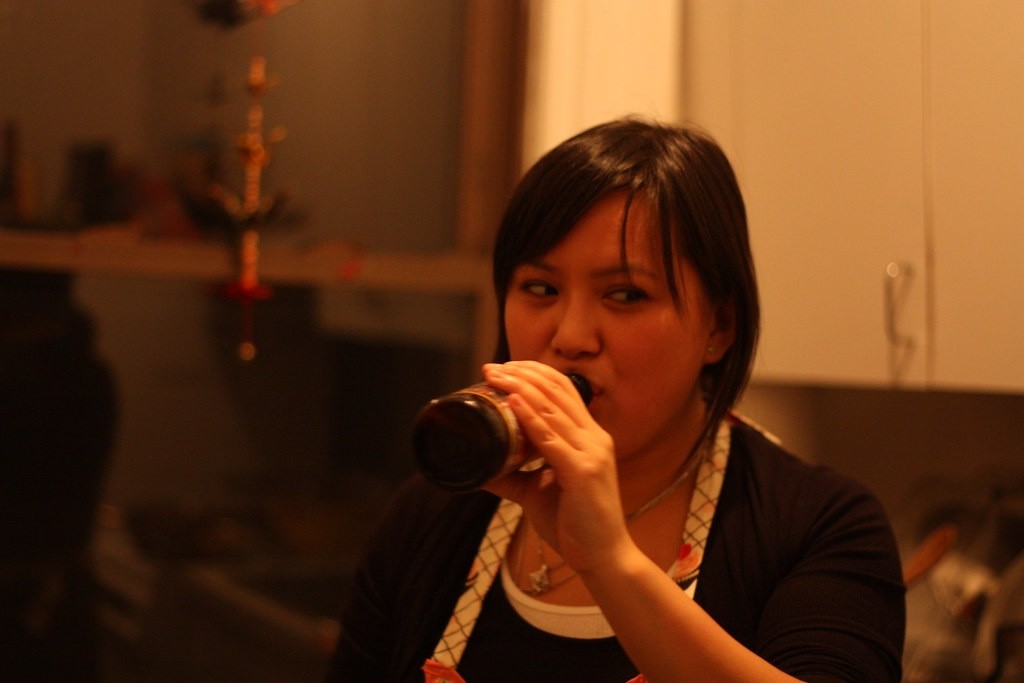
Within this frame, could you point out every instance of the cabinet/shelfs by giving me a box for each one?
[677,0,1024,395]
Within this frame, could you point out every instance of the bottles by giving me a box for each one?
[410,373,592,494]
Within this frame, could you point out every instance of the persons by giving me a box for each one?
[328,119,908,683]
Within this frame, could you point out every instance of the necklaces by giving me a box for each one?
[515,453,702,596]
